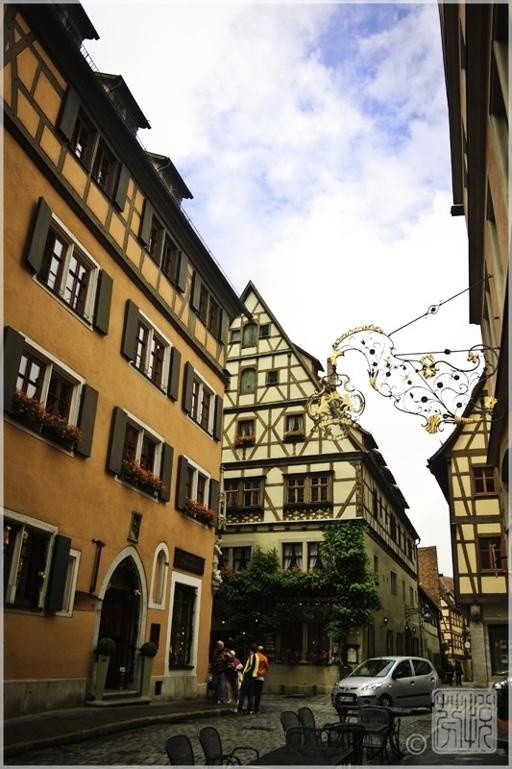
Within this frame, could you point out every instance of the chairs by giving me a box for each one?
[160,697,404,766]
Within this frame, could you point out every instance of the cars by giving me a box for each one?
[330,655,439,716]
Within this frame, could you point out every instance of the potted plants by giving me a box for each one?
[138,642,157,697]
[94,637,115,701]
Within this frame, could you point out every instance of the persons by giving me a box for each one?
[253,645,269,714]
[230,643,260,714]
[445,662,454,686]
[226,649,244,707]
[455,660,462,687]
[211,640,234,705]
